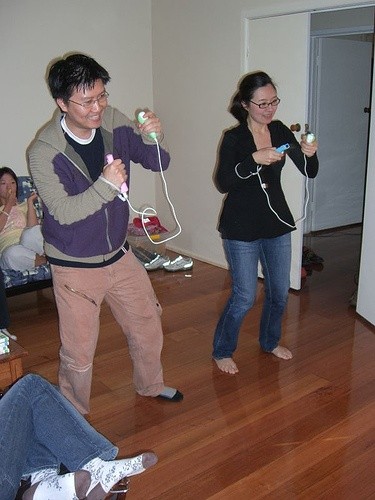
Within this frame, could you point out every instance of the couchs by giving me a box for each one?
[0,174,55,296]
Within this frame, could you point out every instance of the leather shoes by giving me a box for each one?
[304,245,324,263]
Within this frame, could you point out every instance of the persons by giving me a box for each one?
[0,264,17,342]
[0,370,160,500]
[211,70,322,375]
[1,166,52,276]
[23,51,185,416]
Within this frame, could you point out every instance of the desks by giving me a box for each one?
[0,331,30,397]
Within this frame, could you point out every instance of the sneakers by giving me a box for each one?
[162,256,193,273]
[144,255,169,271]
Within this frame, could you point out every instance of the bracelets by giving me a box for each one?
[1,210,10,217]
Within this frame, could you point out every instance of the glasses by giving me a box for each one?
[65,90,110,107]
[246,98,279,108]
